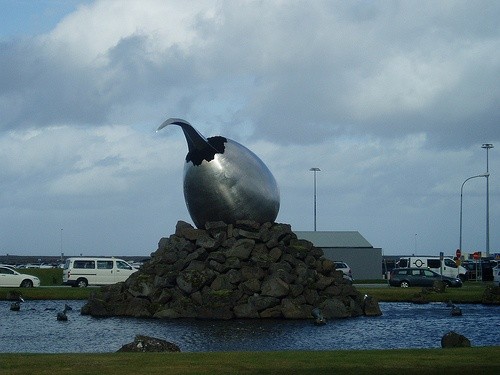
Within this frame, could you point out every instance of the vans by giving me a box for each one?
[62,257,139,288]
[395,256,470,283]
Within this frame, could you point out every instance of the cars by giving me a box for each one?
[0,266,41,288]
[0,262,65,269]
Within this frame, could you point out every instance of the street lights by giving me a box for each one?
[309,168,320,231]
[481,143,494,256]
[460,174,490,251]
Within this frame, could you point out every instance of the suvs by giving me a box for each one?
[389,267,462,289]
[332,261,352,283]
